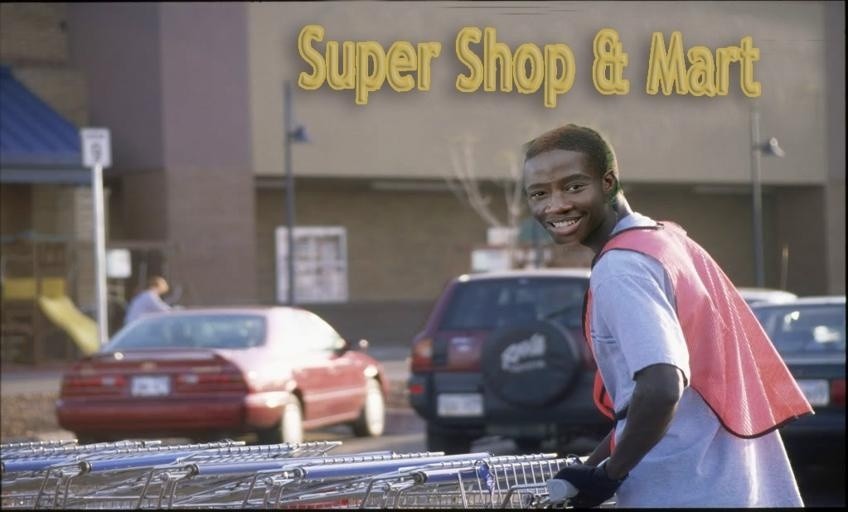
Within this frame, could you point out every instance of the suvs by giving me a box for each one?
[405,266,600,454]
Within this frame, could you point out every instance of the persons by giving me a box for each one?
[520,124,819,509]
[121,277,183,328]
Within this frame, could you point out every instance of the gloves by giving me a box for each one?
[554,460,629,510]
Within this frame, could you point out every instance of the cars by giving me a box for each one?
[729,285,848,472]
[57,307,385,443]
